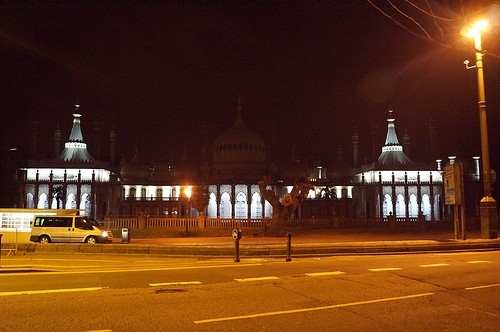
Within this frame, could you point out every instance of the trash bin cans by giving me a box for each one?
[122,228,131,244]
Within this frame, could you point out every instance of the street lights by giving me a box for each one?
[461,18,497,240]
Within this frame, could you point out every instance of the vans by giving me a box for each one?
[30,214,113,244]
